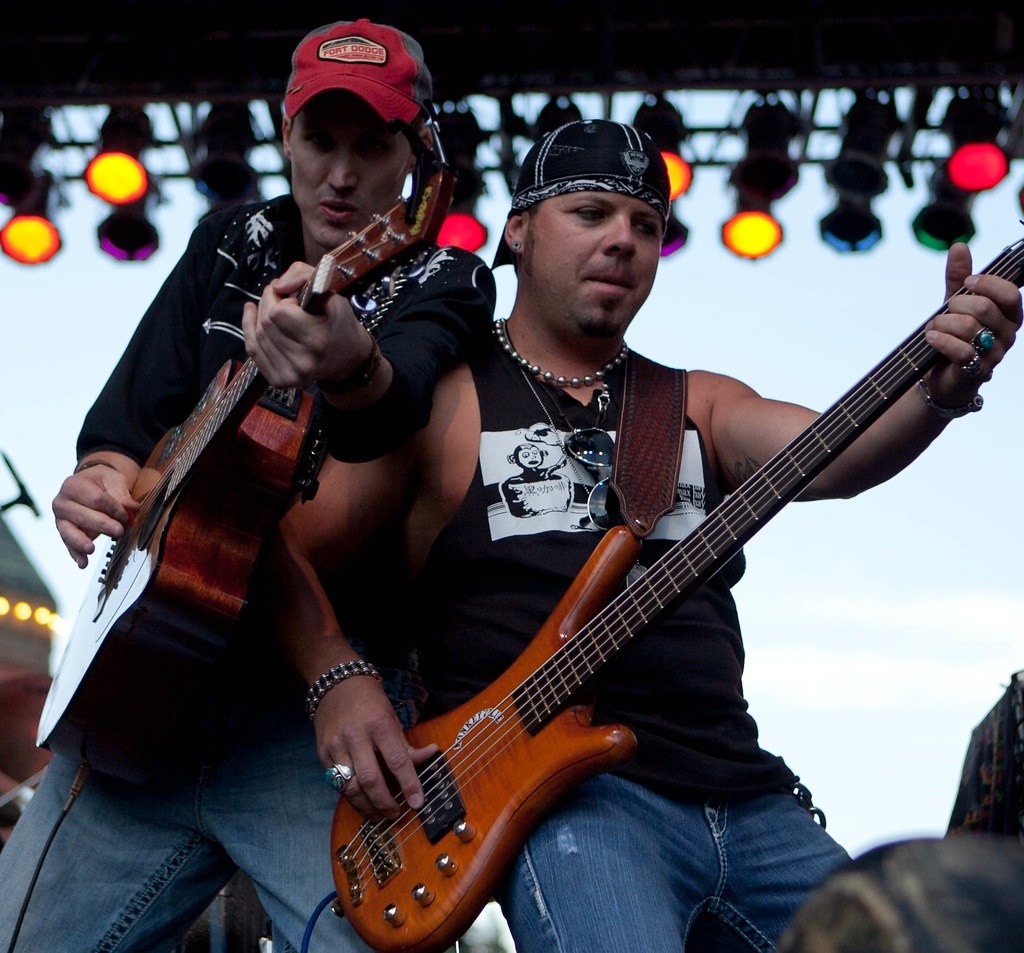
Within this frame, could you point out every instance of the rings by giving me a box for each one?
[324,762,356,794]
[968,323,995,354]
[961,348,984,377]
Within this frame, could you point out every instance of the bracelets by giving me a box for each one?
[73,458,119,475]
[303,658,383,722]
[315,329,382,395]
[916,377,983,418]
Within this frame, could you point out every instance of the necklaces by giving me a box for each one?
[492,316,631,386]
[502,316,650,602]
[502,319,610,484]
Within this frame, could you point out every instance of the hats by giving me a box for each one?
[488,118,671,272]
[285,18,433,125]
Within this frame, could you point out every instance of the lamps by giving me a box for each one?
[1,69,1024,263]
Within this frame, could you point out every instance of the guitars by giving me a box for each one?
[37,173,460,787]
[332,239,1024,951]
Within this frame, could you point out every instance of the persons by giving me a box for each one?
[270,105,1024,953]
[1,18,498,953]
